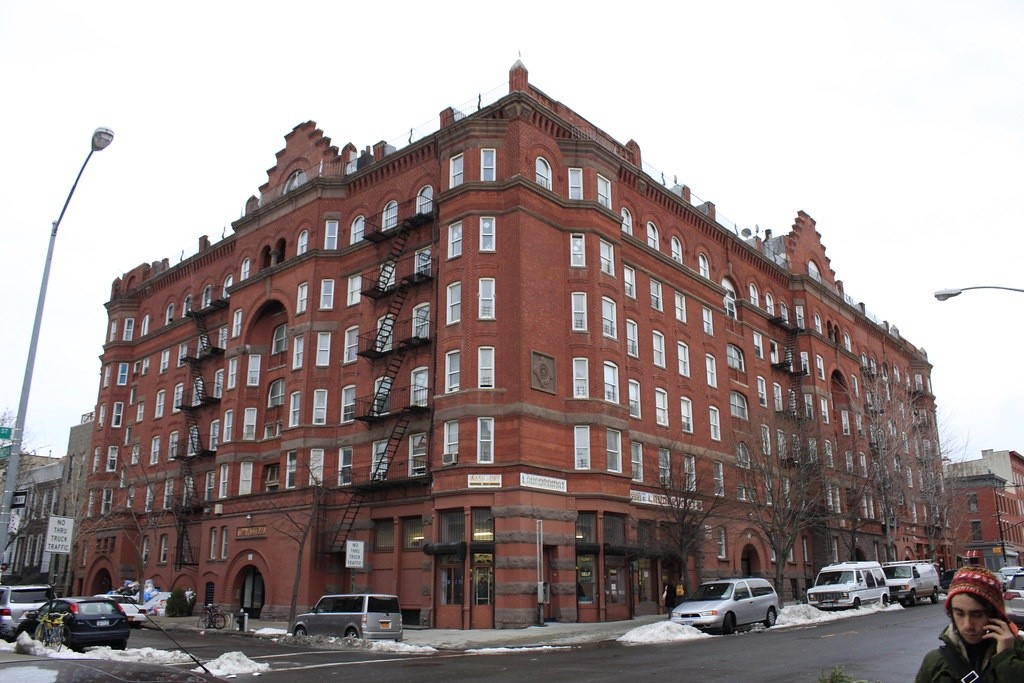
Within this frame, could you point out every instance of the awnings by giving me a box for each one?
[965,551,984,559]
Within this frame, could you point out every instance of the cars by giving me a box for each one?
[994,566,1024,626]
[94,595,147,629]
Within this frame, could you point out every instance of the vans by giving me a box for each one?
[293,594,404,641]
[807,561,891,610]
[881,559,943,607]
[670,577,781,634]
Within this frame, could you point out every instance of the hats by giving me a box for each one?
[945,566,1018,635]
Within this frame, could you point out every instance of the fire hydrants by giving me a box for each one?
[236,613,246,632]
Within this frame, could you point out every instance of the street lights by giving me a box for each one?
[0,127,114,561]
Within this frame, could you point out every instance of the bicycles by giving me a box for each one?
[196,604,230,629]
[34,613,65,652]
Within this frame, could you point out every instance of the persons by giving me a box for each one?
[665,580,677,620]
[914,566,1024,683]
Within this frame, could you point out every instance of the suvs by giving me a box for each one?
[37,595,131,652]
[0,584,58,642]
